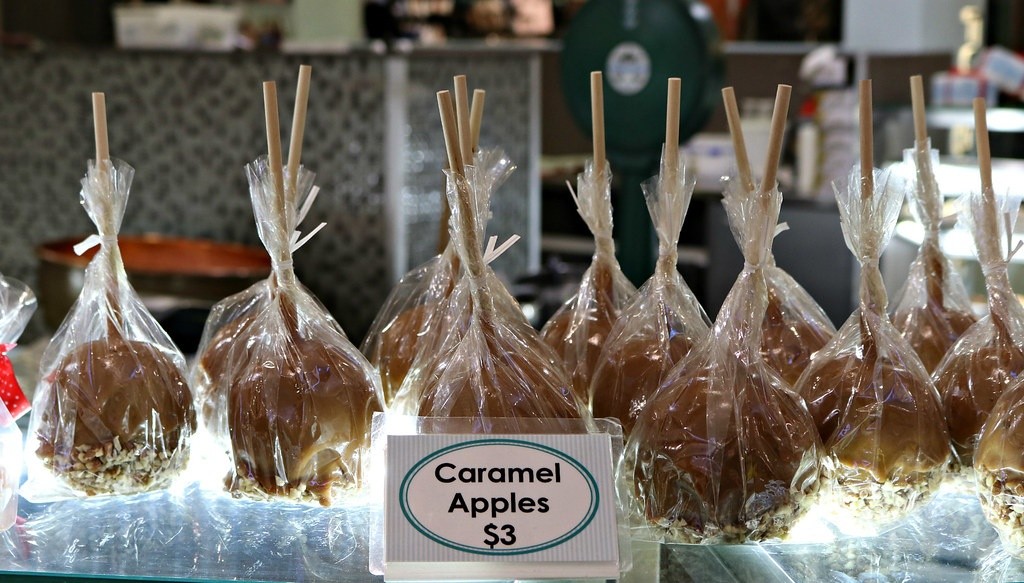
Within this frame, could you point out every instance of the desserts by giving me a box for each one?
[33,290,1024,566]
